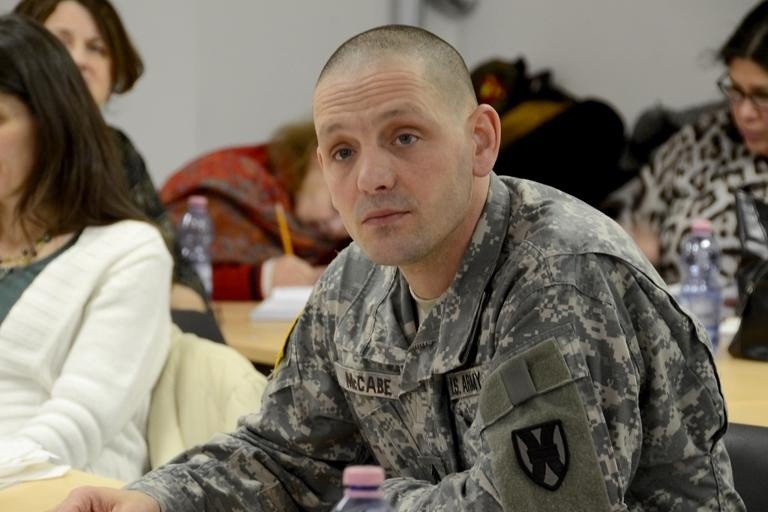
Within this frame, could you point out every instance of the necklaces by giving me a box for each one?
[0,224,50,281]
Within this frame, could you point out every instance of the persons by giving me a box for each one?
[11,1,226,342]
[604,0,768,318]
[47,27,747,512]
[1,15,174,484]
[158,122,353,301]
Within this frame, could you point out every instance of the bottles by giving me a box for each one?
[181,196,213,292]
[331,465,398,512]
[677,219,721,355]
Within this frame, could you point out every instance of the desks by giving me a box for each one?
[0,469,128,512]
[208,299,768,427]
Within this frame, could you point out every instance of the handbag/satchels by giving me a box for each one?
[728,251,768,360]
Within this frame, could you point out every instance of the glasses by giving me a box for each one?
[716,70,767,108]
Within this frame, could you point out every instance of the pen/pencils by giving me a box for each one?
[275,202,292,254]
